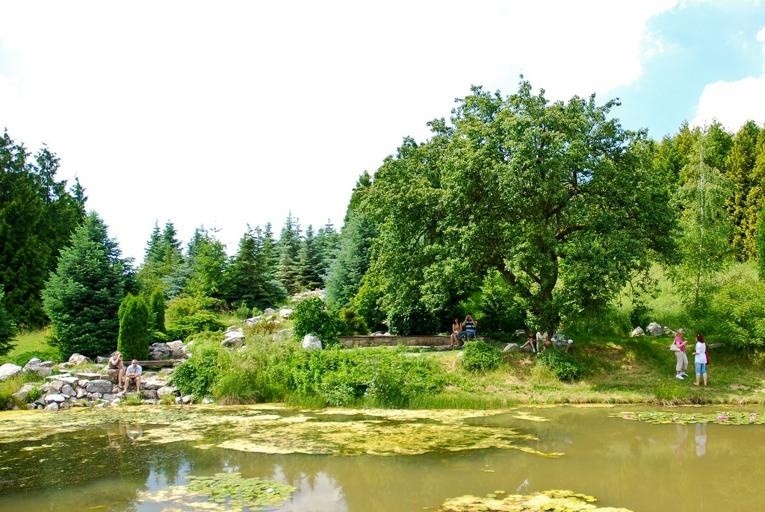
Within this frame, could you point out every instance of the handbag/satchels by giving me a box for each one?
[706,352,710,365]
[670,340,681,352]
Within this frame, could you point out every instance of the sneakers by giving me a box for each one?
[676,372,689,379]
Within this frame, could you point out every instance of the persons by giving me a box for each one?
[122,359,143,394]
[536,328,551,354]
[695,424,707,458]
[123,418,146,450]
[691,333,708,387]
[550,319,572,354]
[105,350,126,390]
[670,424,690,463]
[449,316,462,347]
[458,312,476,347]
[104,410,125,455]
[672,327,689,380]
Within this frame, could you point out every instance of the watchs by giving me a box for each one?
[520,329,536,354]
